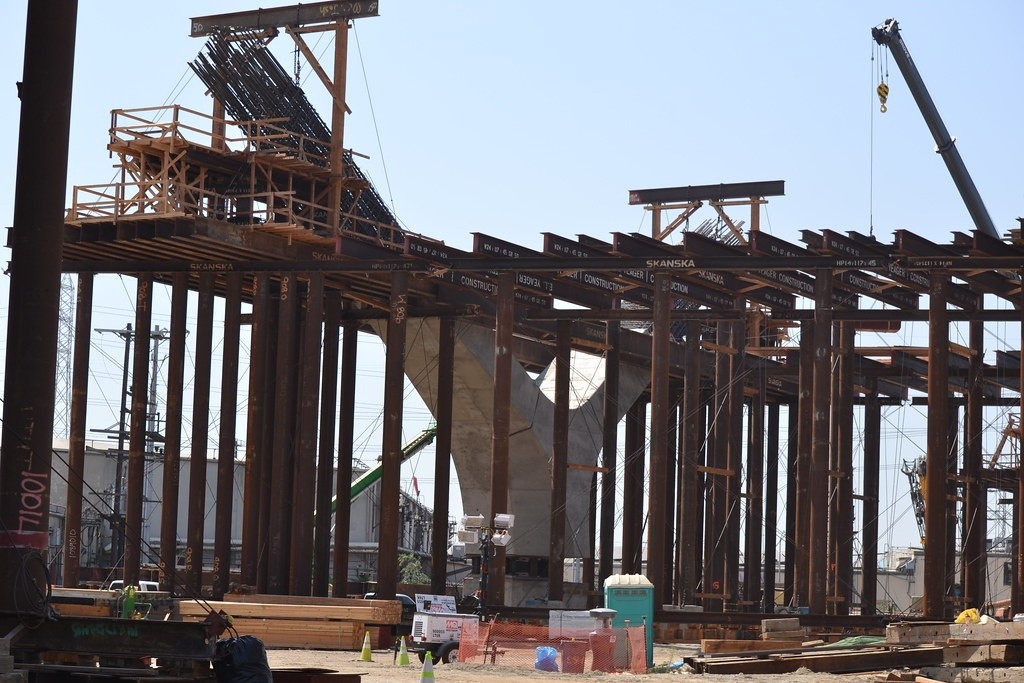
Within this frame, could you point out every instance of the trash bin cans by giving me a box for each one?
[560,639,588,674]
[602,574,655,669]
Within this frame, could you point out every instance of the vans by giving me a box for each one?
[363,592,415,606]
[108,580,159,591]
